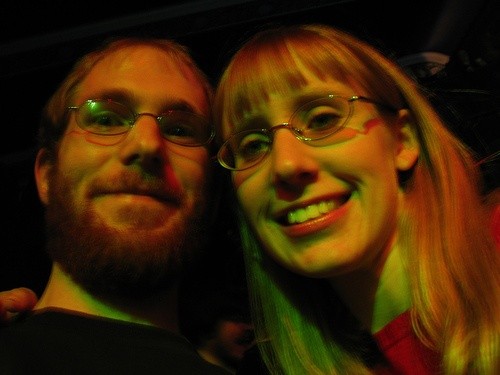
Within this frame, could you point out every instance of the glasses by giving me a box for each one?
[66,97,215,147]
[212,94,381,171]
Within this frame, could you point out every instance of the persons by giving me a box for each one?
[176,257,268,375]
[0,40,232,375]
[2,21,500,375]
